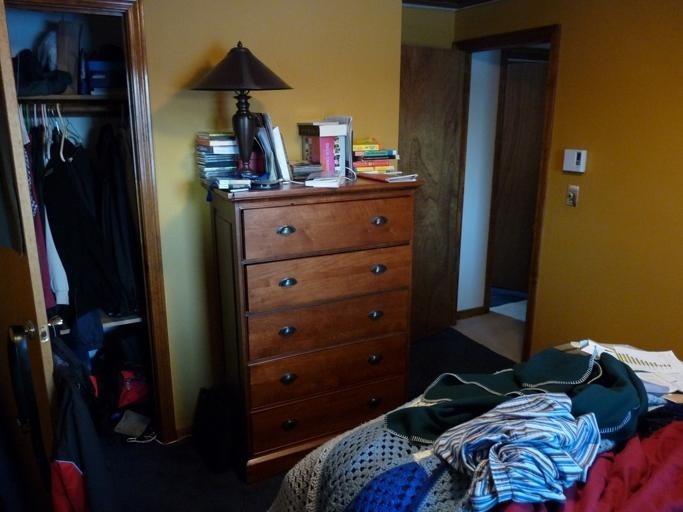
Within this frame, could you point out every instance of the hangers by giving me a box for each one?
[18,103,76,167]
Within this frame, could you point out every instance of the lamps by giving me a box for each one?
[191,38,293,179]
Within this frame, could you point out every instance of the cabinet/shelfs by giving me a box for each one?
[209,181,424,484]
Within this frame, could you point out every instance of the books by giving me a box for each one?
[572,335,683,399]
[190,113,418,191]
[115,408,156,439]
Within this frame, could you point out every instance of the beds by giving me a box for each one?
[264,340,683,512]
